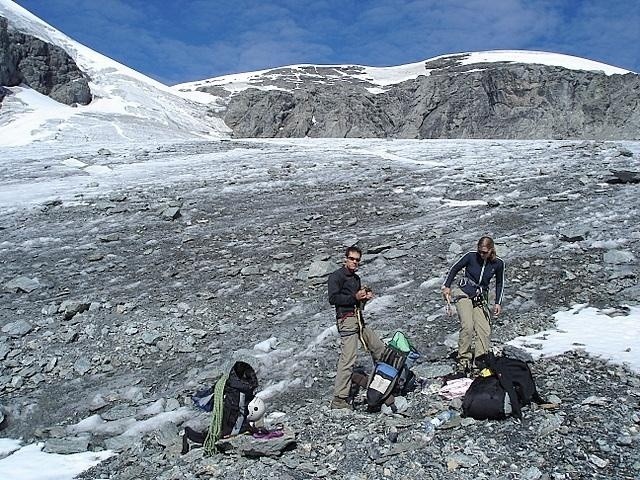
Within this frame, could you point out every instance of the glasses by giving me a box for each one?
[477,248,490,255]
[347,257,360,263]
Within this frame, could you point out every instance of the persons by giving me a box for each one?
[328,247,386,409]
[442,236,505,370]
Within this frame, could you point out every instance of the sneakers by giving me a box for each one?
[457,356,473,376]
[331,396,356,411]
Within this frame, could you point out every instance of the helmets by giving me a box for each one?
[246,395,265,423]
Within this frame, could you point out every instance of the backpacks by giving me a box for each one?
[365,329,422,414]
[458,352,555,423]
[177,360,261,454]
